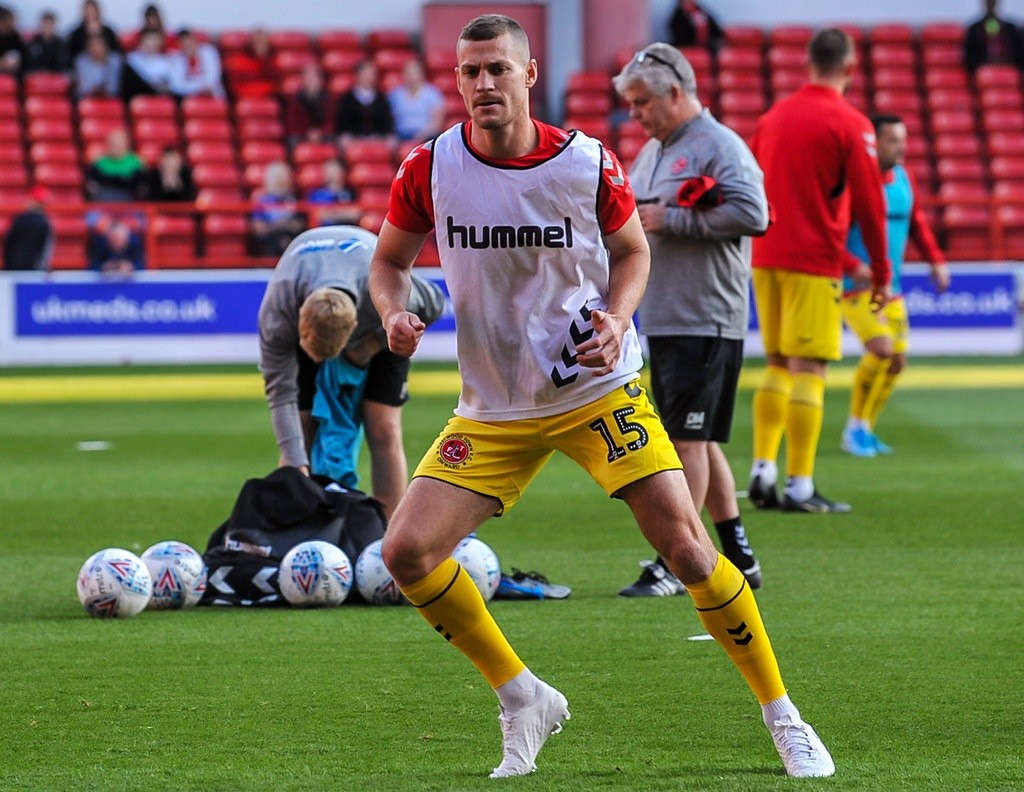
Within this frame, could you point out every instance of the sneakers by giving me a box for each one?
[617,559,688,597]
[748,474,781,510]
[739,558,762,590]
[841,423,890,456]
[781,489,853,513]
[771,713,835,777]
[488,680,571,779]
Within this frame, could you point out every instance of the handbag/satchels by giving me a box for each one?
[197,462,387,611]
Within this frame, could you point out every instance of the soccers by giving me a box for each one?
[355,536,411,605]
[141,540,208,609]
[75,547,153,618]
[451,536,502,602]
[278,538,354,607]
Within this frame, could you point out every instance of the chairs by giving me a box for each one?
[556,21,1024,264]
[0,31,472,270]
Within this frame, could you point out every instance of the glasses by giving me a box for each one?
[635,51,682,81]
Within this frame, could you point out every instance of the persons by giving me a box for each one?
[369,12,835,778]
[259,226,445,532]
[747,26,893,514]
[610,42,768,597]
[1,0,1024,276]
[841,111,951,458]
